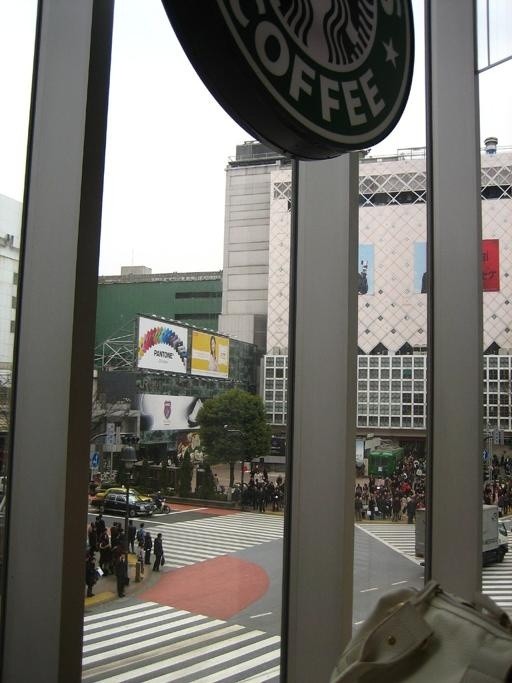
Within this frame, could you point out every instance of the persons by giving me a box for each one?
[221,472,287,512]
[482,448,512,516]
[154,491,164,506]
[84,512,164,597]
[207,335,218,370]
[214,473,219,490]
[354,452,426,522]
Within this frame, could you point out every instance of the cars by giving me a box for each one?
[91,487,154,518]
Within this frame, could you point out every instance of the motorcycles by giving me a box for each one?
[154,499,170,513]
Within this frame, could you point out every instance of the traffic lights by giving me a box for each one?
[122,436,140,445]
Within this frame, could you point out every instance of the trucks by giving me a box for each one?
[416,505,508,565]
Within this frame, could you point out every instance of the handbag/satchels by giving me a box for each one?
[160,554,165,565]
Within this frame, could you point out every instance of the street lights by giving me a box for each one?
[120,446,137,576]
